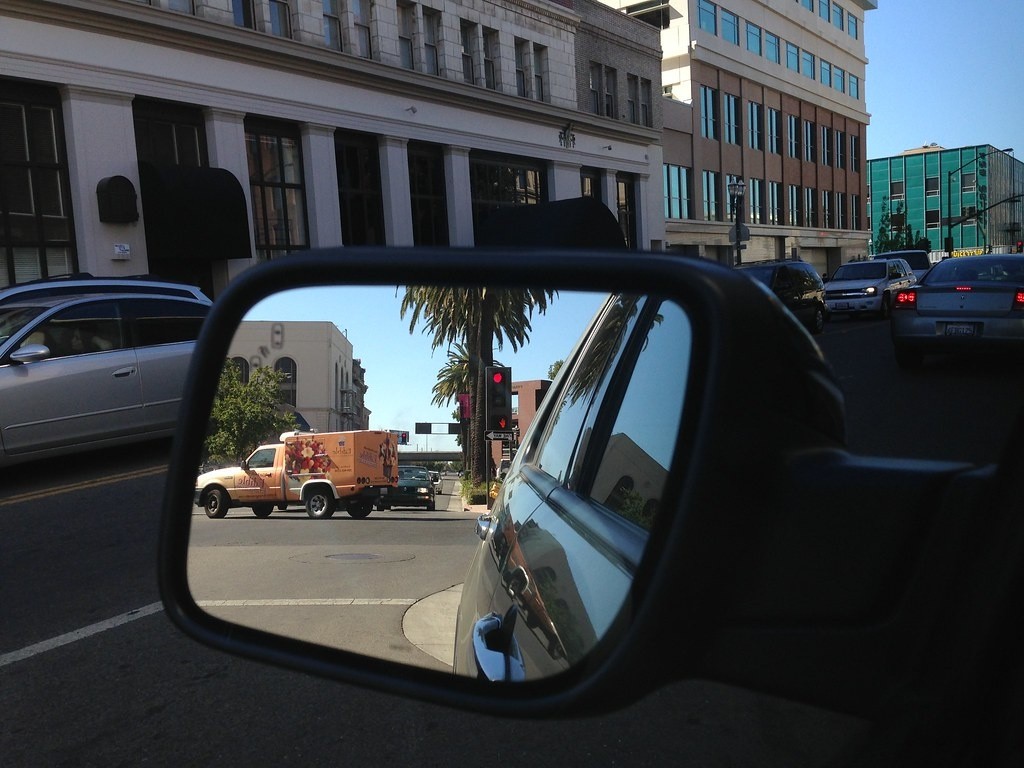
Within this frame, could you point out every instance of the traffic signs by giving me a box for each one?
[485,431,514,442]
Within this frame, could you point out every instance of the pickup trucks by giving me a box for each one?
[196,428,399,520]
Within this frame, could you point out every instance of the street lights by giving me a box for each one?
[947,148,1024,258]
[727,175,747,266]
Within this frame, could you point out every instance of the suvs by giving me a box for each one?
[874,251,933,281]
[0,273,218,467]
[737,257,827,334]
[377,465,439,512]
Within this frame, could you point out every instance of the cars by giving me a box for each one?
[412,470,444,494]
[820,258,921,321]
[889,253,1024,368]
[448,287,692,686]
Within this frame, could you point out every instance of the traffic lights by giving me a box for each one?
[1017,240,1023,252]
[402,433,407,445]
[486,366,513,430]
